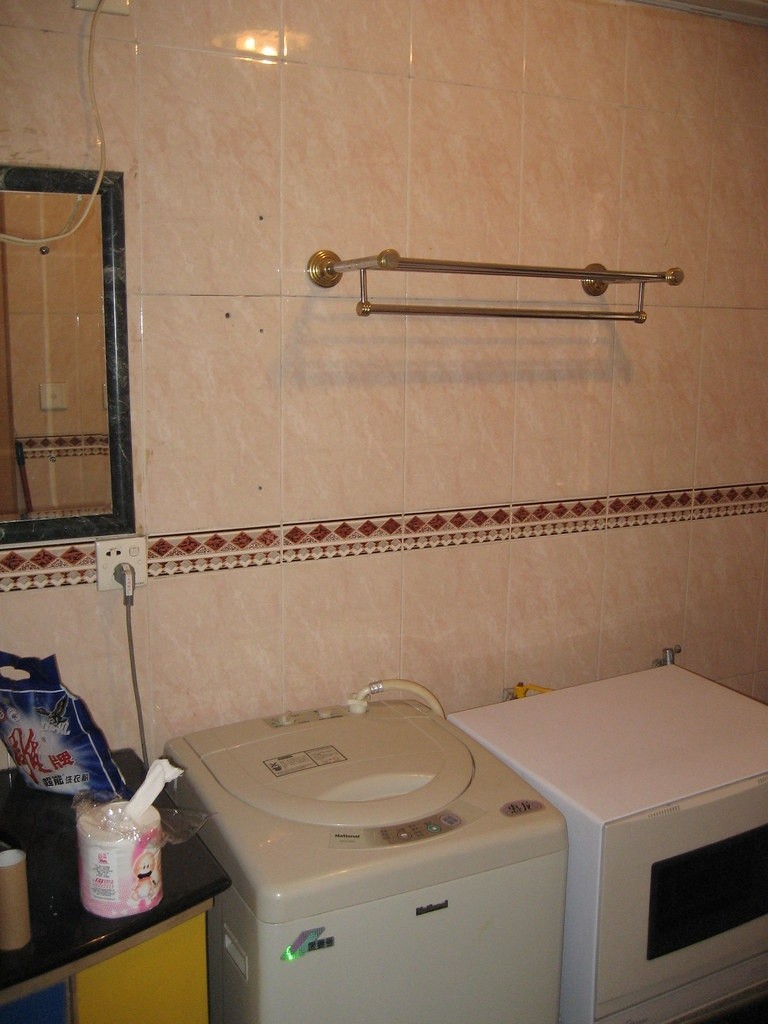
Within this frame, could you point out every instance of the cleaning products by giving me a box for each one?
[0,651,134,804]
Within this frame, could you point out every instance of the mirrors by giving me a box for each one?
[0,164,137,542]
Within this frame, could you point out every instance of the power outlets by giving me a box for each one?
[94,536,148,592]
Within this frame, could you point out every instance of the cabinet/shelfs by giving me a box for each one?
[0,747,233,1024]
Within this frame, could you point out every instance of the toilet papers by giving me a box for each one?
[76,759,184,919]
[0,849,32,951]
